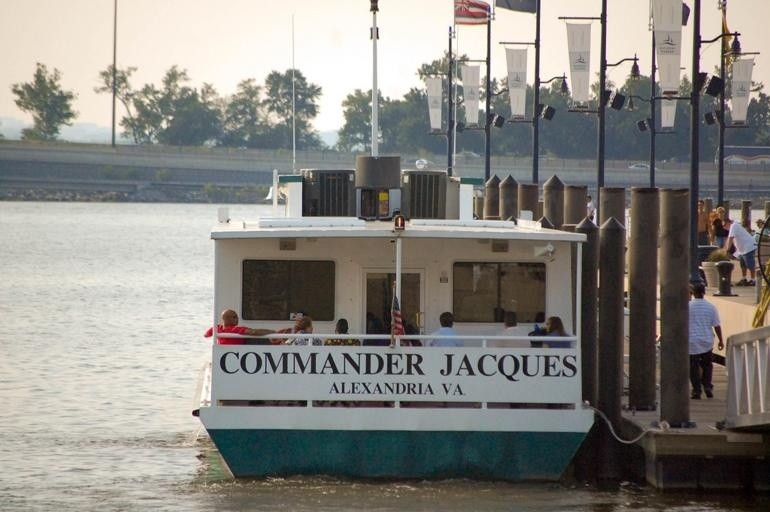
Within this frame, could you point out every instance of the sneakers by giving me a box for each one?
[736,280,756,286]
[689,386,713,399]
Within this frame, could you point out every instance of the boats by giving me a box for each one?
[193,0,596,482]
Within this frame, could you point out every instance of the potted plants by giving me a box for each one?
[698,250,730,288]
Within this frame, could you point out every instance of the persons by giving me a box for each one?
[279,316,322,346]
[424,311,463,347]
[696,200,769,287]
[460,274,497,323]
[203,309,276,345]
[688,282,724,399]
[542,316,571,348]
[496,311,532,348]
[323,318,361,345]
[364,311,424,346]
[586,194,595,222]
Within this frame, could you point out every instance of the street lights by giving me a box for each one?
[533,73,568,185]
[625,96,656,188]
[447,58,509,181]
[597,55,640,227]
[687,33,740,286]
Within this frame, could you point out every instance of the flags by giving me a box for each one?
[495,0,536,14]
[453,0,490,26]
[392,295,405,335]
